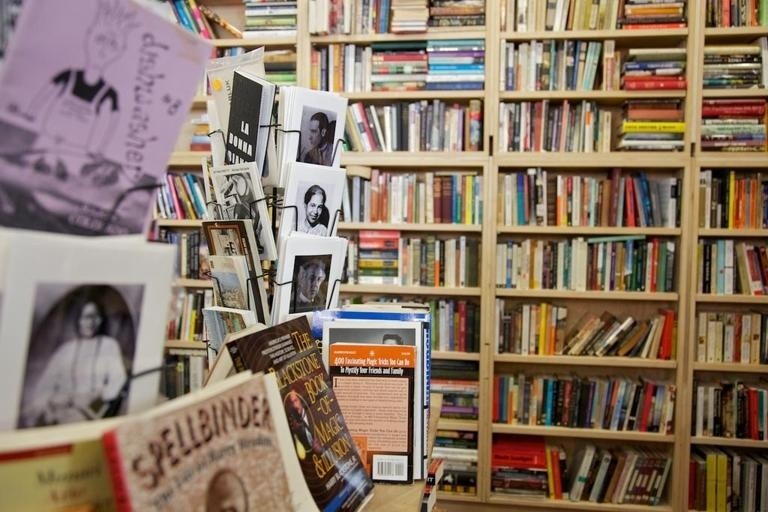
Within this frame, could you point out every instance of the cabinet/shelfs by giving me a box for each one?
[147,1,768,512]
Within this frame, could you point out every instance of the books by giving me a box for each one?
[689,0,767,512]
[1,1,486,512]
[491,1,688,506]
